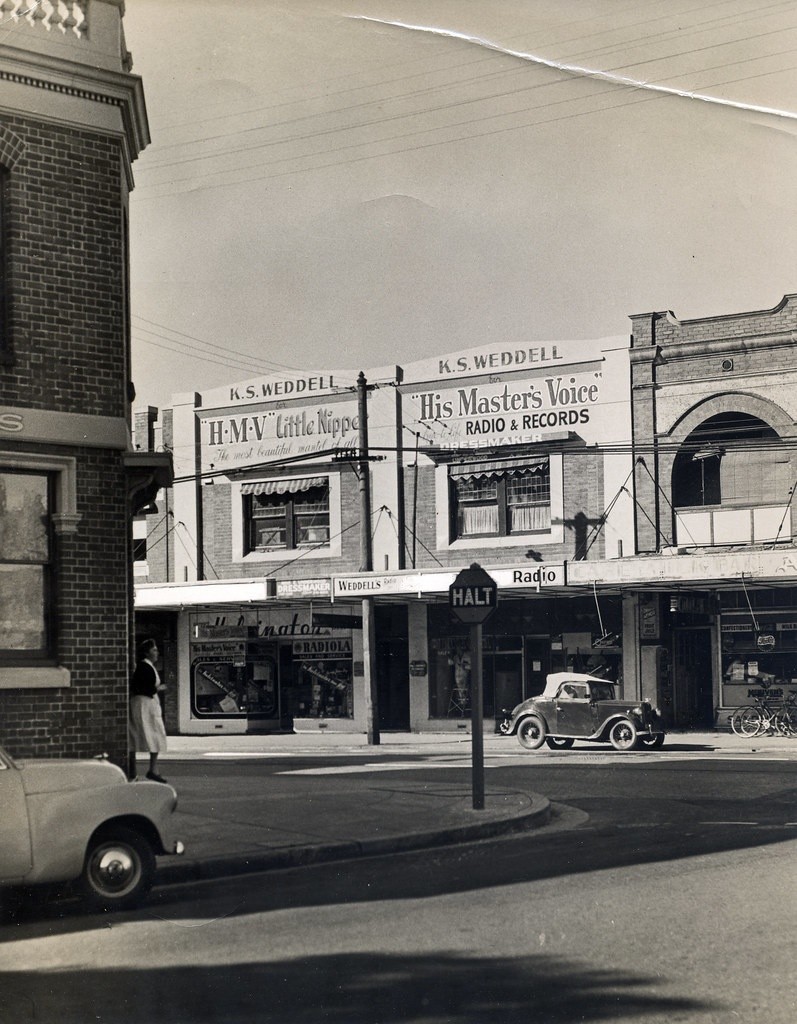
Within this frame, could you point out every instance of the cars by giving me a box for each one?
[501,672,666,751]
[0,747,185,909]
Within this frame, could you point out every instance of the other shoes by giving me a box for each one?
[146,771,166,784]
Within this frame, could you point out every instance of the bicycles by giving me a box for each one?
[731,690,797,738]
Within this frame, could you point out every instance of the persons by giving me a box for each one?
[129,639,168,784]
